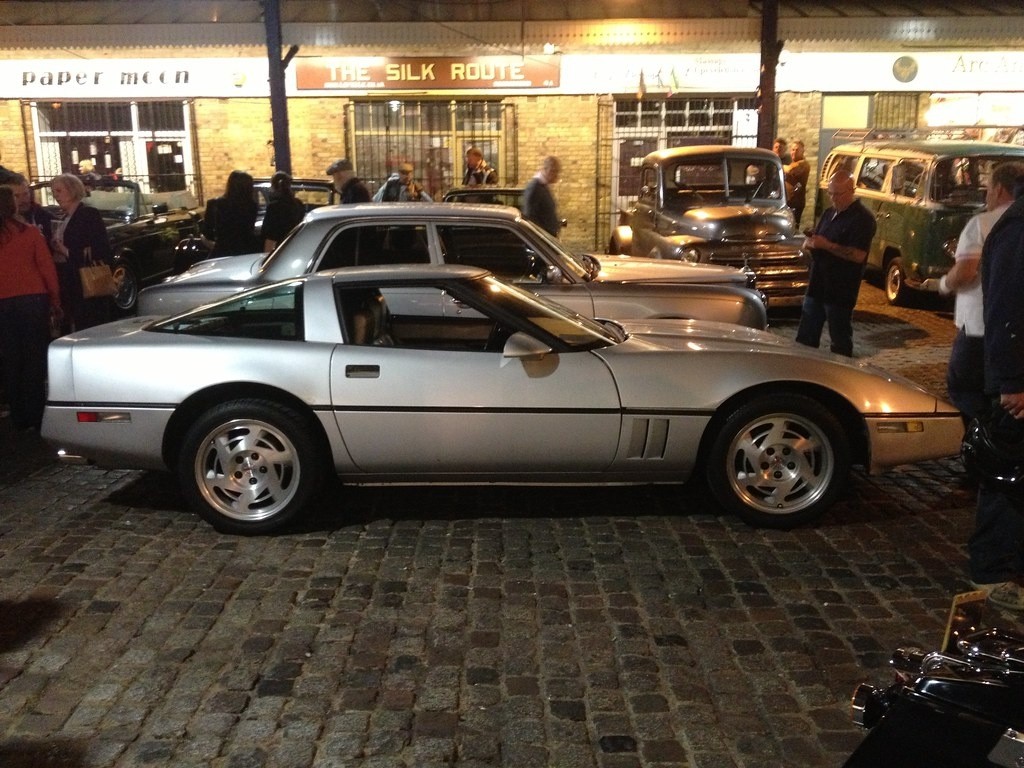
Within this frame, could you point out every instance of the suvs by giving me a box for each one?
[609,144,815,316]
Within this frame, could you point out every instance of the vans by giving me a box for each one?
[813,138,1023,306]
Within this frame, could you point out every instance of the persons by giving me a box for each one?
[262,172,305,252]
[326,158,371,202]
[523,156,562,272]
[795,170,878,357]
[923,161,1024,576]
[0,154,65,452]
[372,163,433,202]
[50,173,117,331]
[202,170,259,259]
[773,138,810,232]
[454,147,499,204]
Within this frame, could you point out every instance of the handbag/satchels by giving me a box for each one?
[959,402,1024,483]
[79,247,118,299]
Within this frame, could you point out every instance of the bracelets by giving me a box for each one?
[940,275,951,295]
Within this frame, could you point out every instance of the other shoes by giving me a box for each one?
[0,404,11,418]
[970,577,1024,611]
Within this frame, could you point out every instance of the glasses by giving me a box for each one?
[829,186,853,196]
[400,170,410,175]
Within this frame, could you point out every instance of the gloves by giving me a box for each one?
[937,276,949,295]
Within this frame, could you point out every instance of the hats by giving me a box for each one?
[326,158,353,175]
[461,148,482,158]
[399,163,414,172]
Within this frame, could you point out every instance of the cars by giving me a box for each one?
[439,185,569,237]
[135,199,769,334]
[208,176,343,258]
[35,264,966,537]
[24,177,217,314]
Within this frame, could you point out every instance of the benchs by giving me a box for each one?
[675,189,735,214]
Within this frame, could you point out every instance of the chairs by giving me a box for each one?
[351,311,375,346]
[370,296,396,344]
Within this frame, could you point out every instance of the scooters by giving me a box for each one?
[829,627,1024,768]
